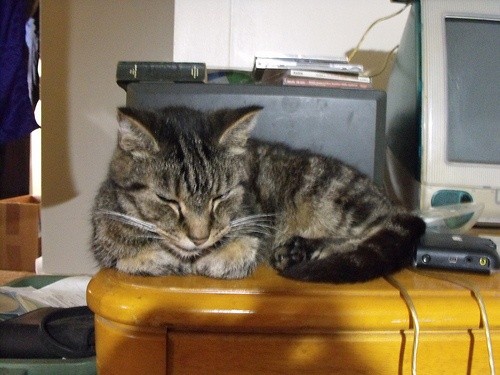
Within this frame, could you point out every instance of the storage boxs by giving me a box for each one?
[2,193,43,274]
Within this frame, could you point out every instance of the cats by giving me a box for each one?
[87,104,428,285]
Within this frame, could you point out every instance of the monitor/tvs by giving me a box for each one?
[384,0,500,226]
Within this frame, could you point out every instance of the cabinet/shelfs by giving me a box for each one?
[85,224,500,375]
